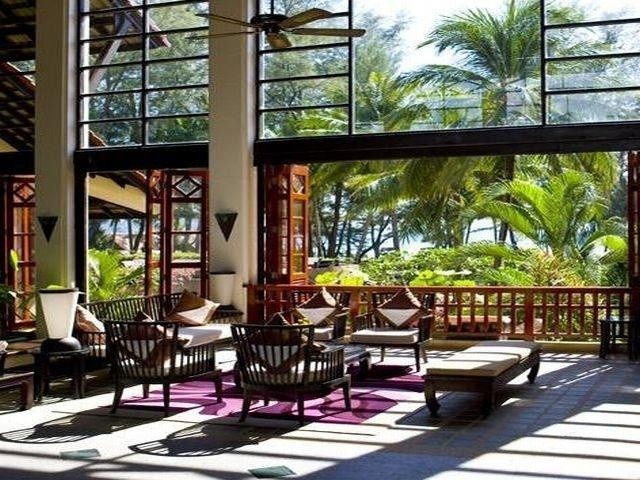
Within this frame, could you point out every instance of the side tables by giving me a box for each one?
[28,347,94,401]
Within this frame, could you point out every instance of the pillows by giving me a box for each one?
[168,291,220,326]
[74,302,107,345]
[113,309,188,366]
[370,285,433,329]
[294,286,341,323]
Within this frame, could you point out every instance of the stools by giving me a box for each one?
[598,314,637,362]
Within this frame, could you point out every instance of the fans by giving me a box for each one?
[185,0,366,48]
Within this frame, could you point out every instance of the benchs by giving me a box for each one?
[419,340,545,418]
[73,291,246,383]
[448,314,512,341]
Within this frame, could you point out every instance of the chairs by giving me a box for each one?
[276,290,351,361]
[350,289,434,375]
[231,320,353,425]
[0,349,35,411]
[101,320,222,415]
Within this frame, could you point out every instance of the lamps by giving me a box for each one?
[36,288,83,351]
[37,216,59,241]
[207,272,243,322]
[214,213,238,241]
